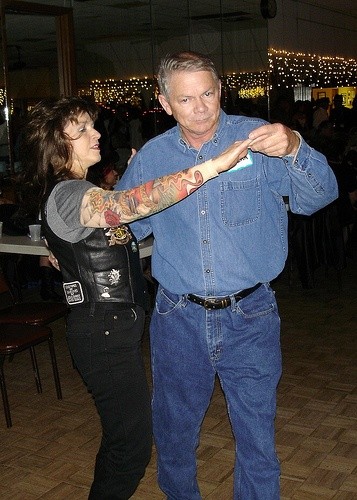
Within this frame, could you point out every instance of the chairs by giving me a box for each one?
[0,267,68,428]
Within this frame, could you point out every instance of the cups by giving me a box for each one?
[29,224,41,241]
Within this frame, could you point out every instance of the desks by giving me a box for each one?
[0,233,158,342]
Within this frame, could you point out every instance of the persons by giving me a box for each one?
[43,97,339,500]
[0,84,357,270]
[19,96,253,500]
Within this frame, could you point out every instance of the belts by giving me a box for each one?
[181,282,263,311]
